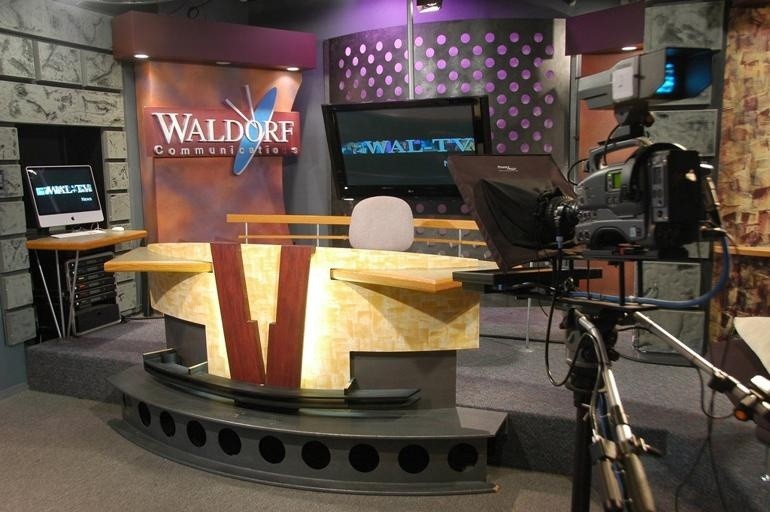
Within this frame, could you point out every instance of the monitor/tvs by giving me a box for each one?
[321,95,490,202]
[24,163,105,228]
[446,152,576,271]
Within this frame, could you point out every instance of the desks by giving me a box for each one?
[25,229,148,344]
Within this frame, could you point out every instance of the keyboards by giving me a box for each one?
[51,229,102,238]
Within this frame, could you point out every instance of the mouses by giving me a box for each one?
[112,227,124,231]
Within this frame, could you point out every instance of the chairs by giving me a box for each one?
[348,195,415,251]
